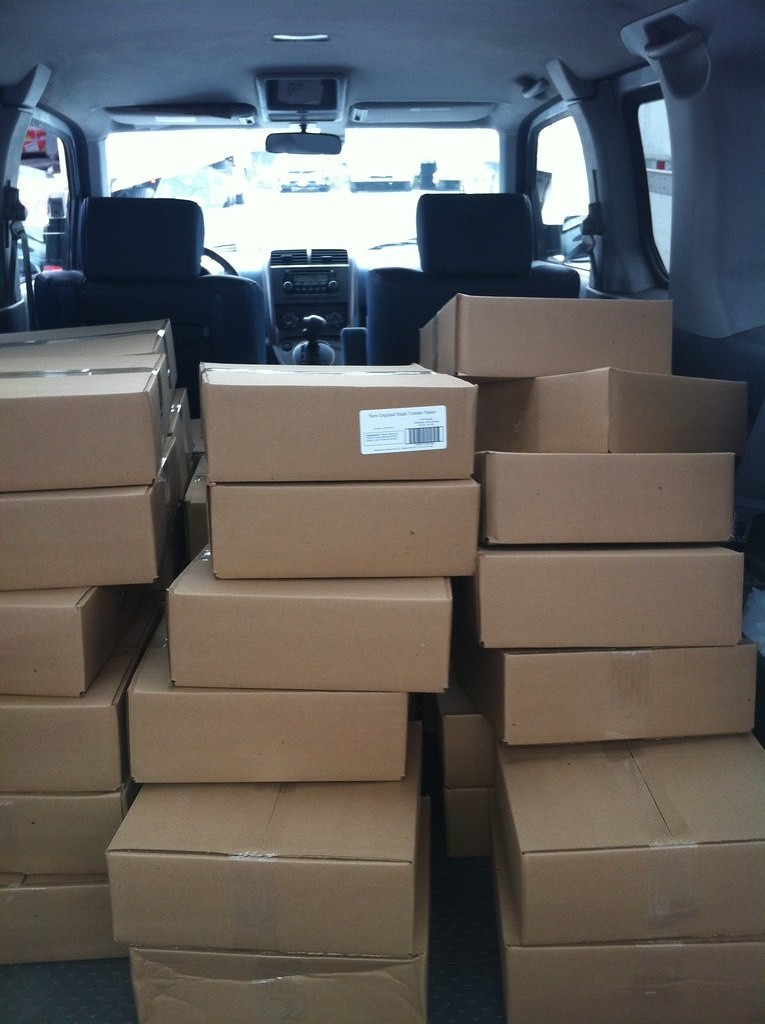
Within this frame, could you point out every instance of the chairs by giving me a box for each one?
[364,192,580,369]
[32,196,266,414]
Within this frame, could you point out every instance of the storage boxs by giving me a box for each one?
[415,290,673,379]
[496,732,765,941]
[208,479,483,581]
[443,786,495,859]
[130,796,433,1024]
[165,546,456,694]
[438,686,495,789]
[462,547,747,647]
[0,602,163,797]
[0,318,207,590]
[474,366,752,454]
[491,823,765,1024]
[475,449,735,545]
[196,358,479,482]
[0,585,150,697]
[106,719,423,956]
[0,776,141,875]
[127,618,409,784]
[0,874,123,965]
[461,642,759,745]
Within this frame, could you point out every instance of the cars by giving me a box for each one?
[274,151,333,191]
[342,138,413,192]
